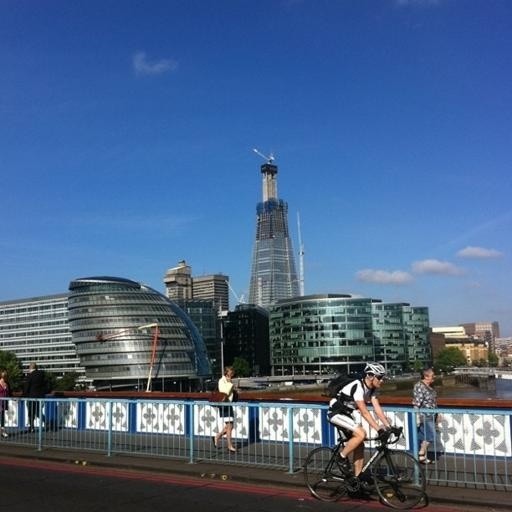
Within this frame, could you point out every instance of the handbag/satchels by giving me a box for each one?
[209,390,228,408]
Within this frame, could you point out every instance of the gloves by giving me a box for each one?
[378,428,388,442]
[389,427,400,436]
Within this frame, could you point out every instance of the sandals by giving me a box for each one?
[416,453,436,465]
[227,447,240,452]
[211,436,218,448]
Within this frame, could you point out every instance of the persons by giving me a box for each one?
[0,367,9,439]
[325,361,394,497]
[23,361,45,434]
[411,368,440,466]
[212,365,236,454]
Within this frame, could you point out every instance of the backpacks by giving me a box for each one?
[325,373,365,398]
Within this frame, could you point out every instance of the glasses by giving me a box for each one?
[373,375,384,381]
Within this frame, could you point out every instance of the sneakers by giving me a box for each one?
[333,452,353,474]
[355,475,377,490]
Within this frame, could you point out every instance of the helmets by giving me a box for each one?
[364,361,386,376]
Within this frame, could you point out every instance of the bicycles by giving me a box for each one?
[303,427,427,510]
